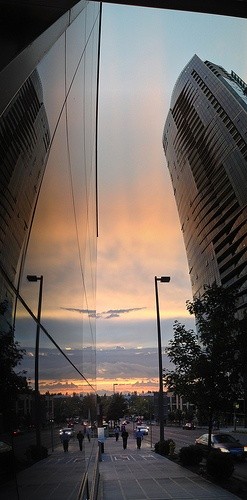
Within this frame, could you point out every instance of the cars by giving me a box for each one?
[121,415,143,425]
[66,416,97,429]
[59,428,75,437]
[183,423,196,429]
[142,424,149,431]
[133,425,148,435]
[195,432,247,460]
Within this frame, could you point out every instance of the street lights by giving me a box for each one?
[154,275,171,439]
[26,274,44,390]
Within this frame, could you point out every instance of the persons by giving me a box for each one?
[114,427,119,441]
[136,428,143,449]
[77,431,84,451]
[61,431,70,453]
[86,428,92,442]
[121,429,128,449]
